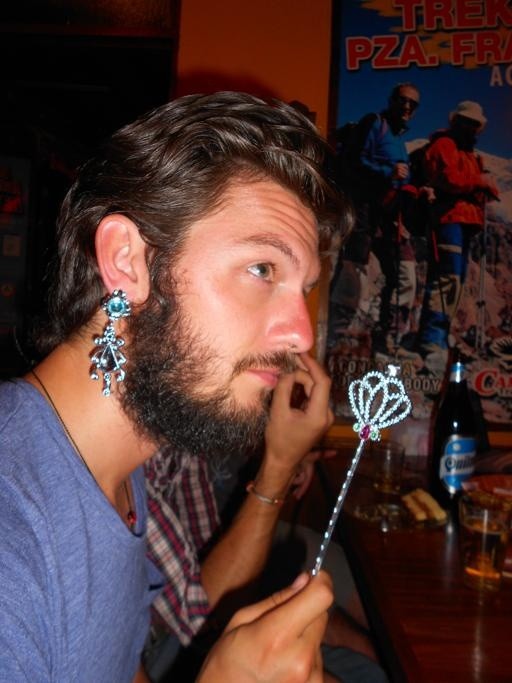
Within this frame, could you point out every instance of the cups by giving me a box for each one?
[373,440,405,498]
[459,492,512,594]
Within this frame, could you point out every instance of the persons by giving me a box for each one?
[0,86,337,683]
[214,446,364,624]
[332,82,437,368]
[404,99,503,367]
[144,352,394,683]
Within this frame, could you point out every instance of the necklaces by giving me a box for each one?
[30,368,137,536]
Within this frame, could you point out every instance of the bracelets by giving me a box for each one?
[244,479,289,507]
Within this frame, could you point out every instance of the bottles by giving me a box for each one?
[429,354,476,510]
